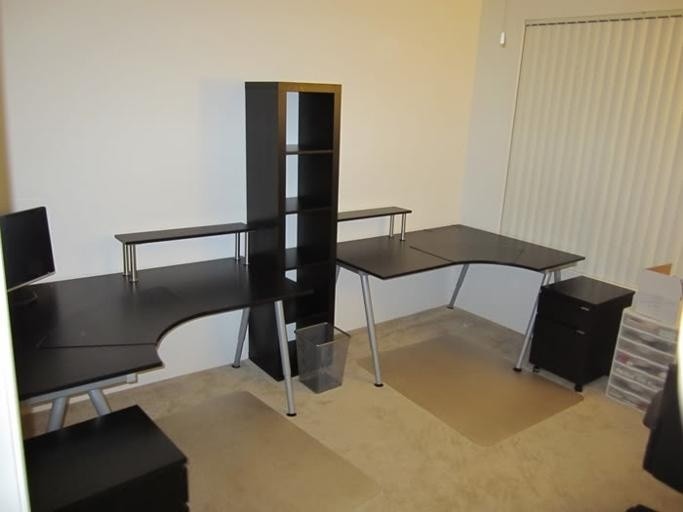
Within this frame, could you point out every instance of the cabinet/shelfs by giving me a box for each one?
[245,78,343,380]
[527,276,634,393]
[603,306,679,415]
[23,403,192,508]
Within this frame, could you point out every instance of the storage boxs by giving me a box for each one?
[635,264,681,328]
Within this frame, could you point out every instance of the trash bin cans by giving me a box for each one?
[294,322,351,394]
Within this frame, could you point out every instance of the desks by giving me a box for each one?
[12,256,318,433]
[336,224,586,388]
